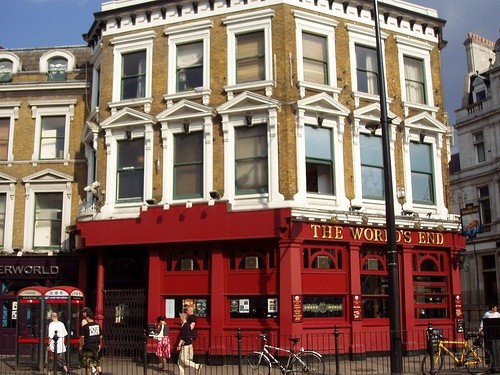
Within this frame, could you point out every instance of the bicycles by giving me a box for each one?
[243,333,325,375]
[420,327,494,375]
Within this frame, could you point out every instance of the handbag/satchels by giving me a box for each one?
[473,335,483,345]
[156,337,171,359]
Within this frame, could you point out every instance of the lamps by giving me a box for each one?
[209,191,221,200]
[330,210,338,223]
[351,205,362,211]
[244,115,253,126]
[126,130,131,140]
[146,199,157,205]
[358,210,368,224]
[435,219,444,232]
[420,130,426,143]
[183,121,191,135]
[13,248,21,254]
[365,122,380,135]
[404,210,413,215]
[412,212,421,229]
[453,248,466,271]
[318,117,323,126]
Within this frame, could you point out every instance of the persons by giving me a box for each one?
[177,313,202,375]
[81,308,90,326]
[185,305,196,340]
[153,316,171,370]
[47,313,69,375]
[479,303,500,346]
[465,219,479,236]
[79,312,103,375]
